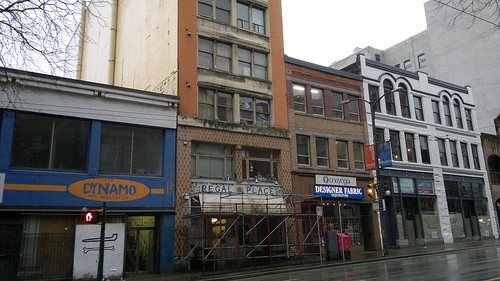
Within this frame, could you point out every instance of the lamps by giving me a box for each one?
[184,194,189,201]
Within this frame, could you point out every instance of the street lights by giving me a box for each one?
[340,87,405,255]
